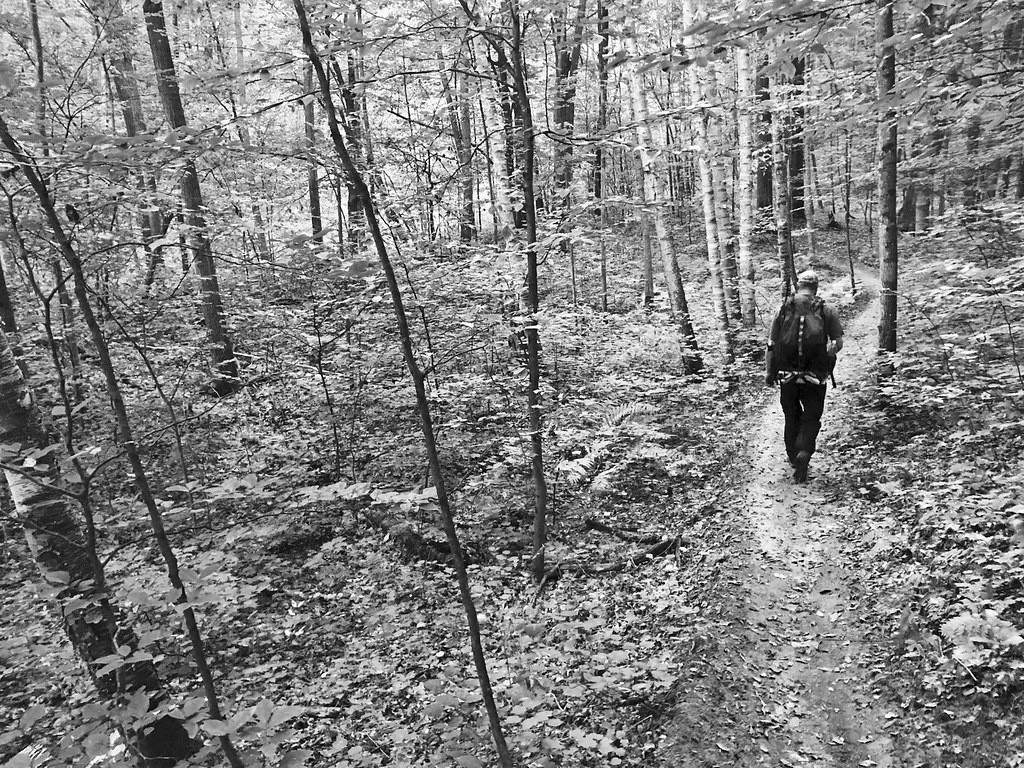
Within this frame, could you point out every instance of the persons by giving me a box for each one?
[765,270,844,483]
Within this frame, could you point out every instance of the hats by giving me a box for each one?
[798,270,818,283]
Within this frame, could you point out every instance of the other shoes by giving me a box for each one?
[793,452,811,484]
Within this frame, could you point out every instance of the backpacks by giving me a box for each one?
[776,296,828,372]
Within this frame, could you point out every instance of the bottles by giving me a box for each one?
[827,340,837,356]
